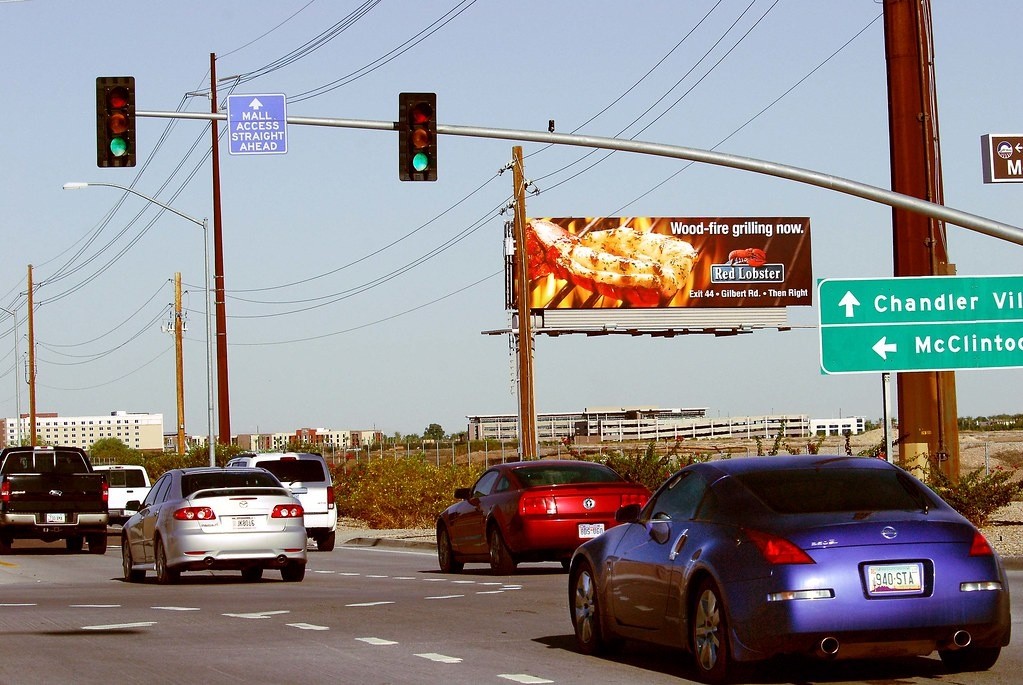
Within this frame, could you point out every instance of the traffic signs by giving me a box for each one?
[225,93,289,155]
[821,320,1023,375]
[814,275,1023,322]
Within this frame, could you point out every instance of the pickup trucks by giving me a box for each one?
[0,446,109,555]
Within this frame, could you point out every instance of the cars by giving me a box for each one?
[435,459,654,575]
[568,455,1013,685]
[122,466,307,583]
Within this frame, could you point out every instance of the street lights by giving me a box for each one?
[61,181,217,466]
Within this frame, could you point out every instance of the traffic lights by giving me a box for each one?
[95,75,135,168]
[399,92,439,183]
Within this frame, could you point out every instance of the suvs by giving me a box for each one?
[223,451,339,551]
[92,464,152,528]
